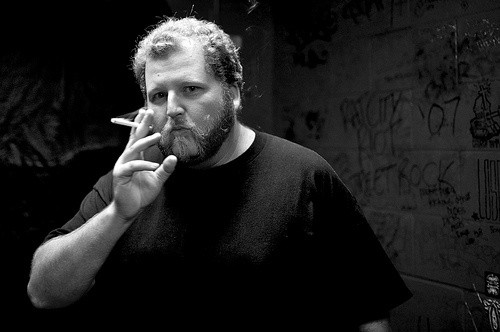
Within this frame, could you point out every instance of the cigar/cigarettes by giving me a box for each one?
[110,117,154,130]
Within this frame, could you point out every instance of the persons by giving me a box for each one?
[26,18,413,332]
